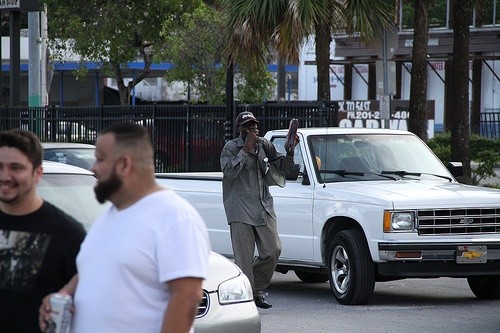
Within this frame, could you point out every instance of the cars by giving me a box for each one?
[22,121,261,333]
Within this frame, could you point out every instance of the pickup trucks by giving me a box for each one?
[151,127,500,305]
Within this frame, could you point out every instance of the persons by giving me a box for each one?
[37,123,210,333]
[0,128,87,333]
[219,111,301,309]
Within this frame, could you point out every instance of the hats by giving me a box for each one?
[235,111,259,126]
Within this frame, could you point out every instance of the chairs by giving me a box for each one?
[338,157,375,172]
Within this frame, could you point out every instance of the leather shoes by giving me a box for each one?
[253,296,272,309]
